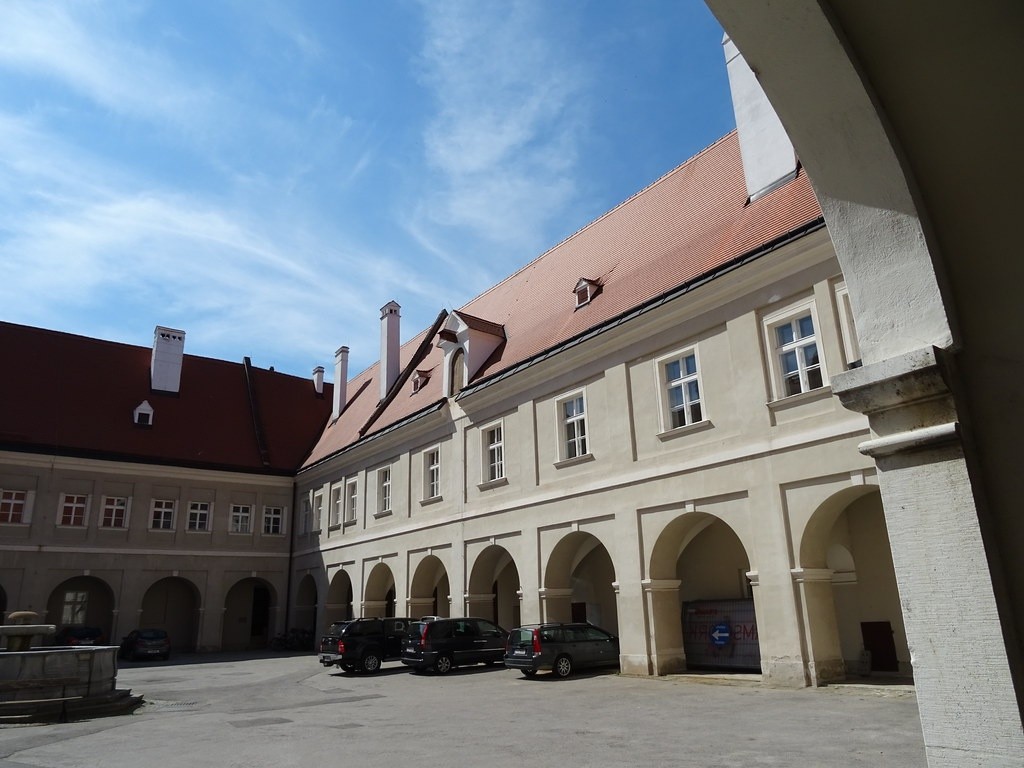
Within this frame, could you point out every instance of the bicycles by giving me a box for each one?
[268,628,314,653]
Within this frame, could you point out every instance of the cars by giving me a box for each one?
[118,628,171,661]
[53,626,104,647]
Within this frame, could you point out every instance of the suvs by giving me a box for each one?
[400,616,510,672]
[317,615,419,675]
[503,621,620,679]
